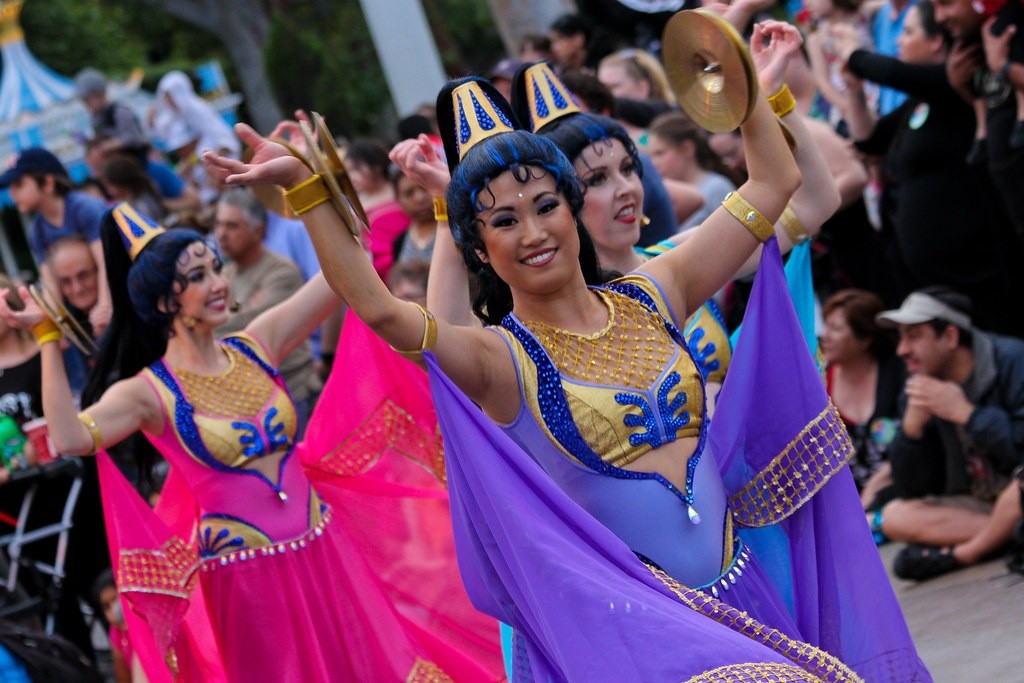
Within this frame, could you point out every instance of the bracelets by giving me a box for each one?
[283,174,331,216]
[433,198,448,221]
[31,320,63,346]
[768,83,797,117]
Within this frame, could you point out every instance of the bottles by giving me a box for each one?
[0,411,27,474]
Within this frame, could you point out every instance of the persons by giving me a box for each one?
[0,109,509,683]
[202,82,933,683]
[96,569,135,683]
[0,1,1024,585]
[388,16,851,622]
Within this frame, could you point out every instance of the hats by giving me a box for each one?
[874,292,972,331]
[62,66,107,104]
[0,148,58,187]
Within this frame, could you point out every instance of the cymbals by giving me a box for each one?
[297,110,371,239]
[29,284,100,356]
[663,9,758,134]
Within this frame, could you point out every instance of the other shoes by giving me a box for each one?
[894,544,962,579]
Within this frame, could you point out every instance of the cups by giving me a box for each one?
[22,419,60,465]
